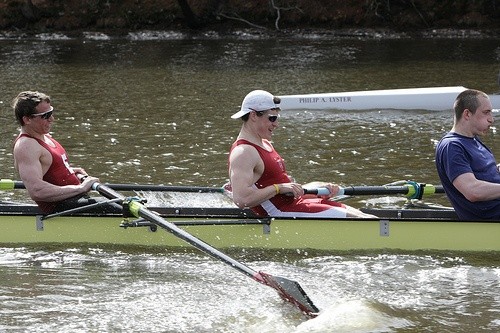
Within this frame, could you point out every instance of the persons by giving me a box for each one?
[11,90,162,215]
[228,90,379,219]
[434,89,500,221]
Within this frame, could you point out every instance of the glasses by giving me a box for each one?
[249,106,280,122]
[32,105,53,119]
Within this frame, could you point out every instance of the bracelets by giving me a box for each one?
[273,184,279,195]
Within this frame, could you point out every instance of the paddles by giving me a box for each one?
[302,176,445,198]
[0,177,223,195]
[94,182,321,318]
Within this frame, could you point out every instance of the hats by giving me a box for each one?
[230,90,281,119]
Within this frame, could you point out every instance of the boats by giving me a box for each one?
[269,86,500,110]
[0,178,499,254]
